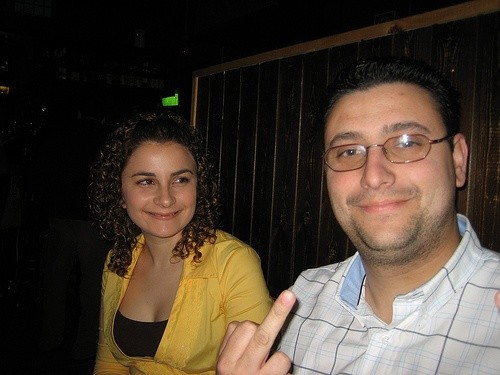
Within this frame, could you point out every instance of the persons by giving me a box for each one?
[91,112,272,374]
[215,59,500,375]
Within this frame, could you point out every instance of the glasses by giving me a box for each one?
[320,133,454,172]
